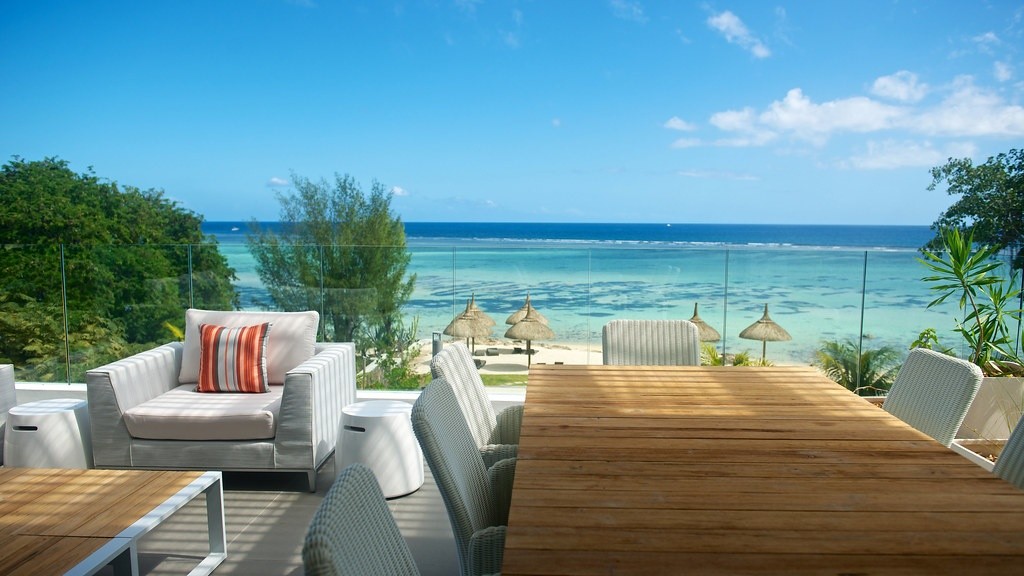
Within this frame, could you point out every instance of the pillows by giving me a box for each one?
[178,308,320,384]
[192,322,273,393]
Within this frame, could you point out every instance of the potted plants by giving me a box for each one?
[907,220,1024,445]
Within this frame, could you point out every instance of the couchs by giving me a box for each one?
[86,341,356,492]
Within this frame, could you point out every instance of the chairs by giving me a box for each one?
[430,340,524,470]
[301,463,420,576]
[603,319,700,366]
[991,412,1024,487]
[881,348,984,449]
[411,376,517,576]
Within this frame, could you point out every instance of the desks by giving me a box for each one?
[500,364,1024,576]
[0,467,227,576]
[336,400,424,498]
[4,398,94,468]
